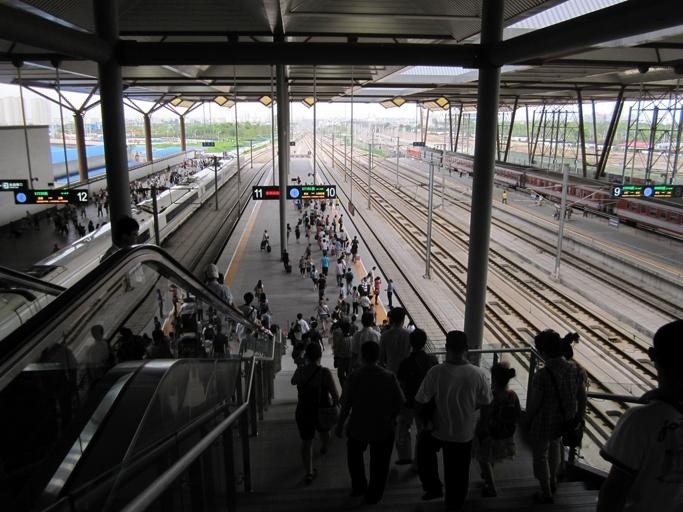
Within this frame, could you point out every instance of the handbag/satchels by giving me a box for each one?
[565,414,584,447]
[319,407,337,428]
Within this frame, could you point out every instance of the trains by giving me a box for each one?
[0,152,245,344]
[406,143,682,242]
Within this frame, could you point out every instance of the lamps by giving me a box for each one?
[162,93,452,111]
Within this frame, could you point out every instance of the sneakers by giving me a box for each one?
[421,492,443,500]
[349,486,367,497]
[534,481,556,505]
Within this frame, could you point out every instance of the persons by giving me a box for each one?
[1,283,251,512]
[216,272,246,316]
[239,279,281,335]
[291,306,588,511]
[205,262,234,307]
[597,202,613,214]
[595,319,683,512]
[292,266,393,337]
[260,174,360,303]
[9,152,228,253]
